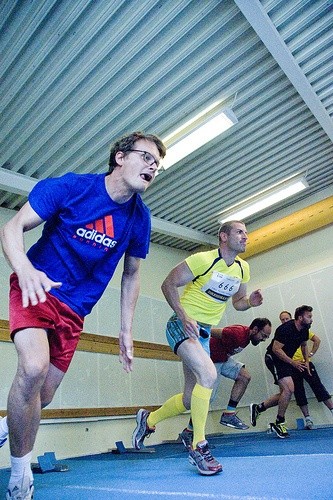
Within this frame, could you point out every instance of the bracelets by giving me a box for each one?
[309,353,313,356]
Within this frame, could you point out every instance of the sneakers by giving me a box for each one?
[178,428,193,452]
[271,419,290,438]
[131,409,155,451]
[304,418,313,430]
[249,403,260,427]
[0,416,7,448]
[218,410,249,430]
[5,467,33,500]
[188,440,222,475]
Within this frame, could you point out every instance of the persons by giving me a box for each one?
[131,220,263,476]
[279,311,333,430]
[249,305,313,438]
[178,318,271,452]
[0,131,167,500]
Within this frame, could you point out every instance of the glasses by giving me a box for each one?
[124,149,165,174]
[257,330,269,339]
